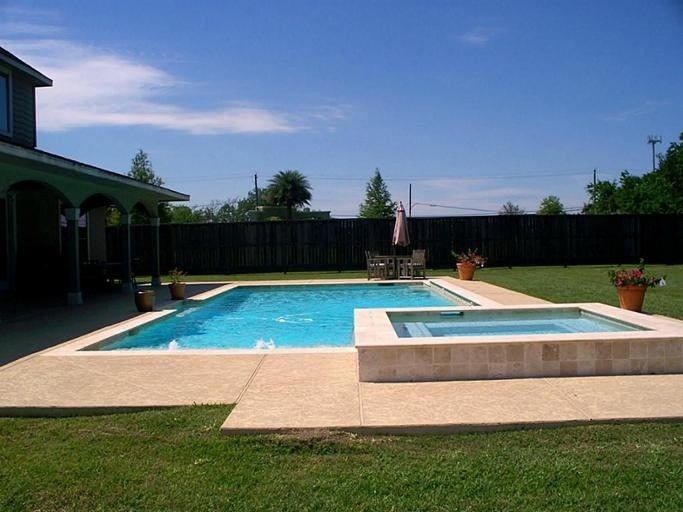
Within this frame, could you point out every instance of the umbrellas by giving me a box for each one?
[391,201,411,249]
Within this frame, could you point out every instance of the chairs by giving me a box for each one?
[365,250,426,281]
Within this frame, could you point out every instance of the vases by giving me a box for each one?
[617,286,647,313]
[457,263,476,280]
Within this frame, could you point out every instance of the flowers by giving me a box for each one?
[607,258,668,288]
[451,247,488,268]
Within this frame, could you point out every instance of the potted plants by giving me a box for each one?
[134,267,187,312]
